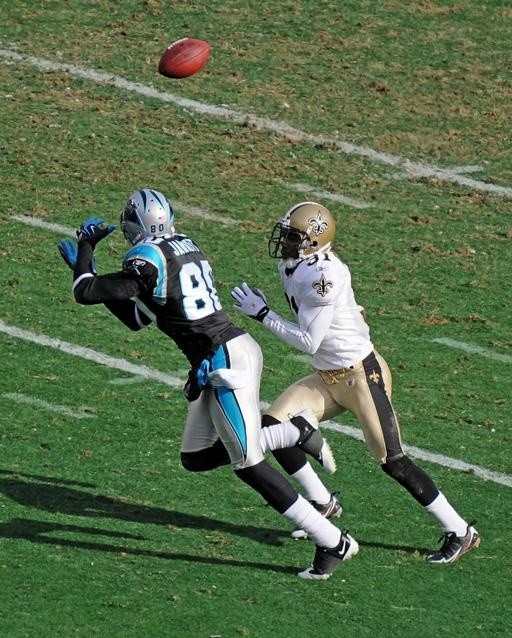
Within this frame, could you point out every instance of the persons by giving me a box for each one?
[57,188,360,580]
[230,202,481,564]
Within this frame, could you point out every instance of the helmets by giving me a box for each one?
[119,189,174,246]
[269,202,336,263]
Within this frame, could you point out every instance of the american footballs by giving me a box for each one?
[157,39,210,77]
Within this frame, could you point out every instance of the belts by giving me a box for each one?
[317,366,354,385]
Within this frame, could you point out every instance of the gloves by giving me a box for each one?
[57,238,95,268]
[231,281,269,322]
[75,218,115,248]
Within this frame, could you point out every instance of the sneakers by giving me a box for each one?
[298,528,359,580]
[291,491,343,540]
[427,520,481,564]
[287,408,337,475]
